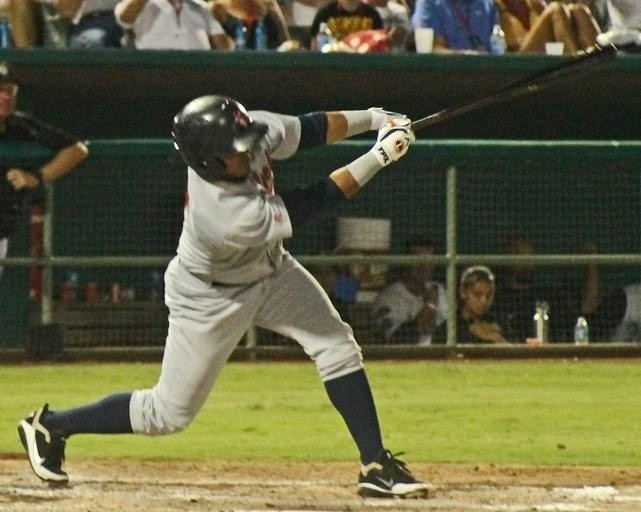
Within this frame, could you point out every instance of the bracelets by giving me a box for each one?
[30,169,45,189]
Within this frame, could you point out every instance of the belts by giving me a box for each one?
[210,281,249,290]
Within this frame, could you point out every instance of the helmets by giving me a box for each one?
[172,95,269,183]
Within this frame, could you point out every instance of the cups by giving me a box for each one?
[546,42,564,56]
[414,27,434,53]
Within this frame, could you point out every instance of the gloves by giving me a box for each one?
[368,107,416,169]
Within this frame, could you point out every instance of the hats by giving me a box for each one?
[0,60,20,83]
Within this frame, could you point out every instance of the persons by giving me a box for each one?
[1,0,641,55]
[16,94,433,499]
[258,232,640,345]
[1,65,88,346]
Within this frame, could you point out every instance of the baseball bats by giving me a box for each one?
[411,42,618,139]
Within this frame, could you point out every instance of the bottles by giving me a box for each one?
[232,21,248,49]
[315,22,332,52]
[575,312,588,345]
[489,24,505,55]
[0,19,10,48]
[253,17,267,49]
[532,302,552,341]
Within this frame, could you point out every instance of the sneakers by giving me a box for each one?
[357,448,430,500]
[17,403,72,483]
